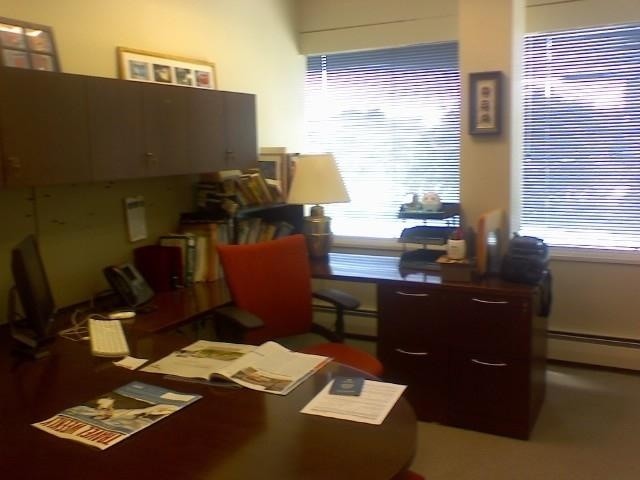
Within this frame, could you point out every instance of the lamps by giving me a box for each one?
[286,154,351,259]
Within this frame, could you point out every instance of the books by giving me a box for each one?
[157,216,294,289]
[29,379,205,451]
[192,146,298,211]
[139,339,334,396]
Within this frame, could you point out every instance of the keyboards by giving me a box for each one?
[88,319,130,358]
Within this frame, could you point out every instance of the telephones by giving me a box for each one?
[103,263,154,309]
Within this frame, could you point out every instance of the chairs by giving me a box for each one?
[214,204,400,391]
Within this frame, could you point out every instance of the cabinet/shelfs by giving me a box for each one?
[0,71,257,189]
[378,263,548,442]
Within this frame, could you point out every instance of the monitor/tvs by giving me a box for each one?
[7,233,57,349]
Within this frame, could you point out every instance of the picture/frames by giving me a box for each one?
[0,17,63,72]
[469,71,502,135]
[118,46,217,89]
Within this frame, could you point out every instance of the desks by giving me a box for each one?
[0,321,419,480]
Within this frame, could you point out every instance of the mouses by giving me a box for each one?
[109,311,135,318]
